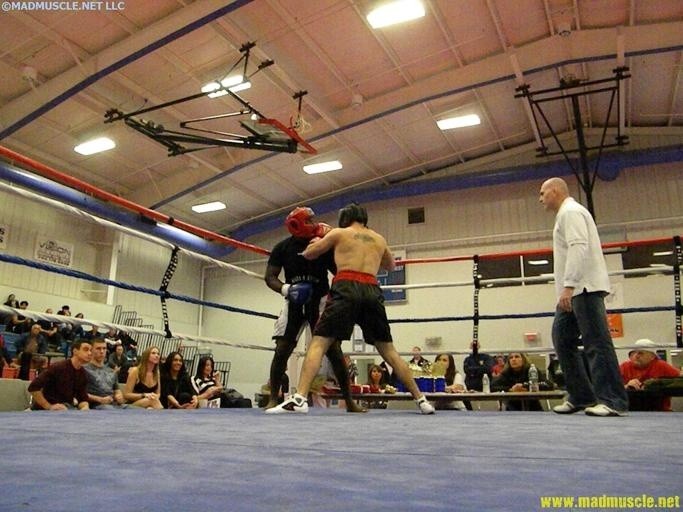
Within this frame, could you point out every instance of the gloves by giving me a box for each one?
[315,222,334,238]
[288,283,313,305]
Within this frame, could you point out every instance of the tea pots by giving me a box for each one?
[370,384,379,392]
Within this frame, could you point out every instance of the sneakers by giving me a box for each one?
[265,393,309,414]
[553,400,596,414]
[414,395,435,414]
[584,403,629,417]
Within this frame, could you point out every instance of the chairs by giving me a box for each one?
[318,355,683,413]
[1,324,230,413]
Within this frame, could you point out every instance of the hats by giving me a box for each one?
[629,338,662,360]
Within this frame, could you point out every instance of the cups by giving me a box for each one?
[362,383,369,392]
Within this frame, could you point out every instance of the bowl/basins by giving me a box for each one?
[320,383,362,394]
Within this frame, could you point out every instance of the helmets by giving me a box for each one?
[285,207,318,237]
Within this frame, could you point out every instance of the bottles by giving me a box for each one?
[528,364,538,392]
[397,362,447,392]
[28,368,36,381]
[481,373,491,393]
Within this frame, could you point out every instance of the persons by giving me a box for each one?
[343,339,539,412]
[539,178,629,417]
[617,340,683,412]
[265,203,436,415]
[0,294,237,411]
[264,208,369,413]
